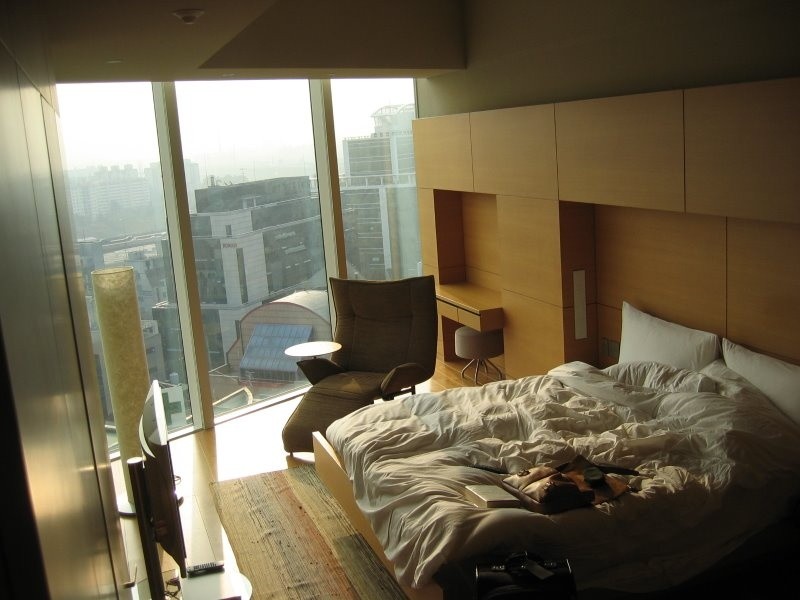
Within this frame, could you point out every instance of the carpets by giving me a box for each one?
[208,461,410,600]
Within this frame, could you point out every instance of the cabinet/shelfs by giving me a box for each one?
[0,41,138,600]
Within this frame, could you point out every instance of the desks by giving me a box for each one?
[284,341,342,358]
[436,280,504,332]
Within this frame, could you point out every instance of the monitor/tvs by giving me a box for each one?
[138,380,188,580]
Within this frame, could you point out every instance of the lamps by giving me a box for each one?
[90,265,184,518]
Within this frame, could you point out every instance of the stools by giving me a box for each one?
[455,326,504,385]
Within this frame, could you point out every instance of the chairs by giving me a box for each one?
[283,275,438,455]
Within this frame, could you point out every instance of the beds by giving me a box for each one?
[311,363,800,600]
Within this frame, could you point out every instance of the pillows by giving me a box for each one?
[618,301,722,371]
[721,338,800,425]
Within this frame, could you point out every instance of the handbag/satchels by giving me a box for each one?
[502,454,639,514]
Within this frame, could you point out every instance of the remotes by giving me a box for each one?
[187,561,226,573]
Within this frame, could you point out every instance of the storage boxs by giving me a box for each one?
[464,484,521,509]
